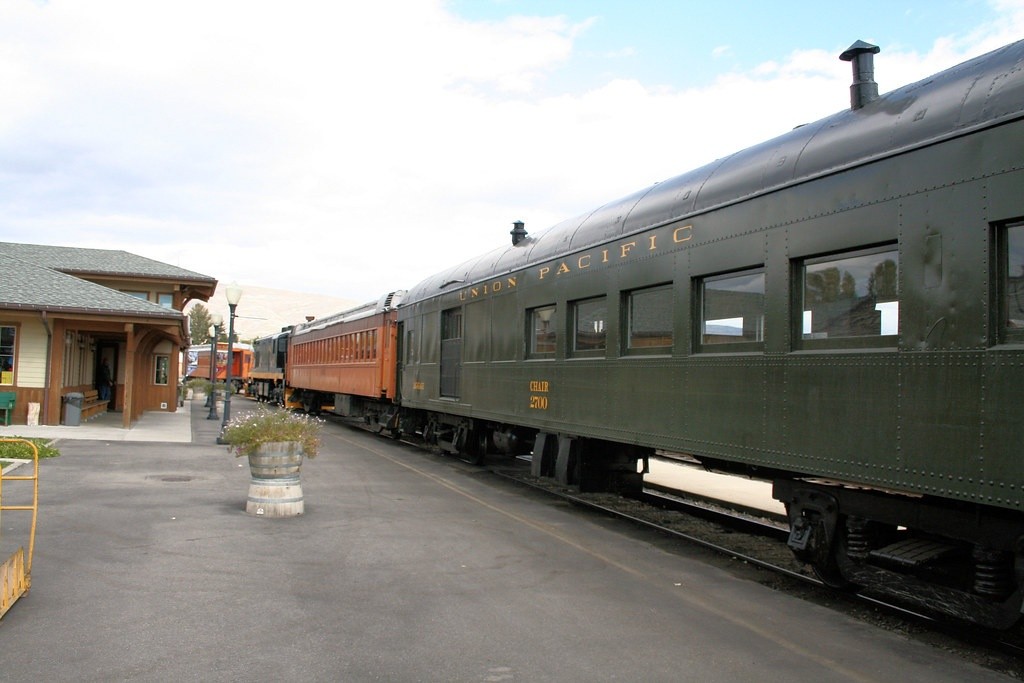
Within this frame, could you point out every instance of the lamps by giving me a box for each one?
[89,342,97,353]
[77,337,85,349]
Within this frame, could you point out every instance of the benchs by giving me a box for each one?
[0,392,16,427]
[63,390,111,422]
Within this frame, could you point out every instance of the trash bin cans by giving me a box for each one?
[61,392,84,426]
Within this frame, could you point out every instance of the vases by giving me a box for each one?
[248,441,304,479]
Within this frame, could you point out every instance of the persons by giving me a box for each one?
[100,358,113,400]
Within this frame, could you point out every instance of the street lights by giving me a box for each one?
[206,312,224,420]
[205,324,215,407]
[216,283,242,445]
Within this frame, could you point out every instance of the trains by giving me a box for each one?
[188,38,1024,659]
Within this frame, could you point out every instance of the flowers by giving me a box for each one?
[220,400,326,458]
